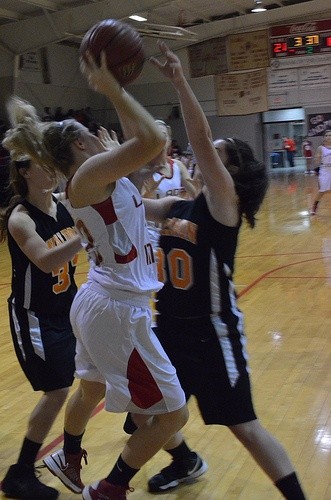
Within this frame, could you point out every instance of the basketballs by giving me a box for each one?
[80,18,145,87]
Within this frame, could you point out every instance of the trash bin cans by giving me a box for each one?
[271,153,280,168]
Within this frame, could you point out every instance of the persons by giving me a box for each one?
[281,135,297,167]
[301,135,317,174]
[0,37,307,500]
[310,129,331,216]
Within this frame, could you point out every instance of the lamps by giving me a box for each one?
[251,0,267,13]
[129,11,150,23]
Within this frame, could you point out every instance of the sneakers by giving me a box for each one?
[147,452,209,494]
[82,478,135,500]
[43,447,88,493]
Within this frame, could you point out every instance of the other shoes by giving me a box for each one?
[310,205,318,214]
[2,464,59,500]
